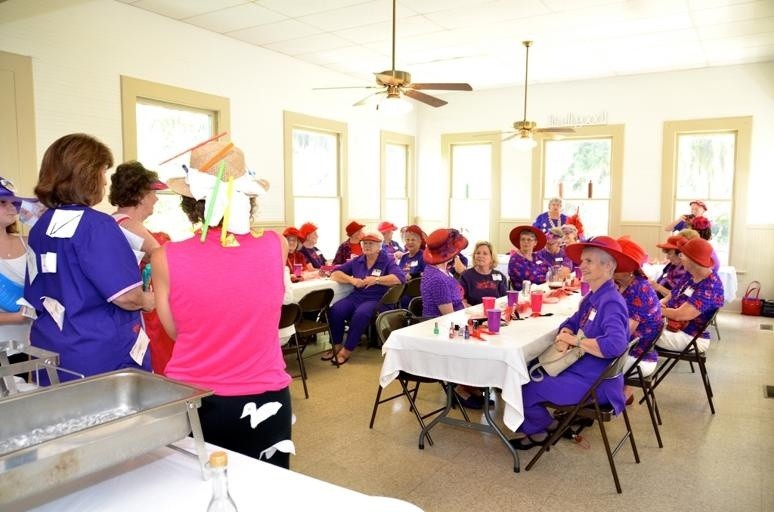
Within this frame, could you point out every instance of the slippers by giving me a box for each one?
[331,352,352,365]
[321,348,341,361]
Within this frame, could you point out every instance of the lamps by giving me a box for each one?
[374,96,413,123]
[511,131,538,152]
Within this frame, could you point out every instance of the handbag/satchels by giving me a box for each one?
[742,281,764,316]
[538,328,589,377]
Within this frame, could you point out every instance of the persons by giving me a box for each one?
[0,177,39,384]
[421,228,508,409]
[24,133,156,386]
[508,199,583,291]
[649,201,724,353]
[283,221,427,365]
[509,235,663,451]
[151,142,296,469]
[109,161,169,272]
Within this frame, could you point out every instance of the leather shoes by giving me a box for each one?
[452,387,495,410]
[509,434,549,450]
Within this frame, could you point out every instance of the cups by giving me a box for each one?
[294,264,303,278]
[546,269,564,289]
[486,309,502,333]
[482,297,496,317]
[580,281,590,298]
[575,267,582,279]
[521,279,532,297]
[530,291,543,313]
[506,290,519,307]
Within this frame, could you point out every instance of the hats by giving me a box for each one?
[166,140,269,200]
[423,228,468,264]
[400,224,427,249]
[509,225,547,252]
[150,179,166,190]
[655,235,714,267]
[360,236,381,242]
[378,221,398,232]
[282,223,318,243]
[564,236,648,274]
[346,221,364,236]
[689,201,707,211]
[0,177,39,204]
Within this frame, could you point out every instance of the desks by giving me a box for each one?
[278,254,512,347]
[0,387,424,512]
[377,263,737,473]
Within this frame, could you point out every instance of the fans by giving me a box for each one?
[312,0,472,107]
[477,41,575,141]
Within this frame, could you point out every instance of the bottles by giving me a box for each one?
[434,319,480,339]
[574,435,590,449]
[206,451,238,512]
